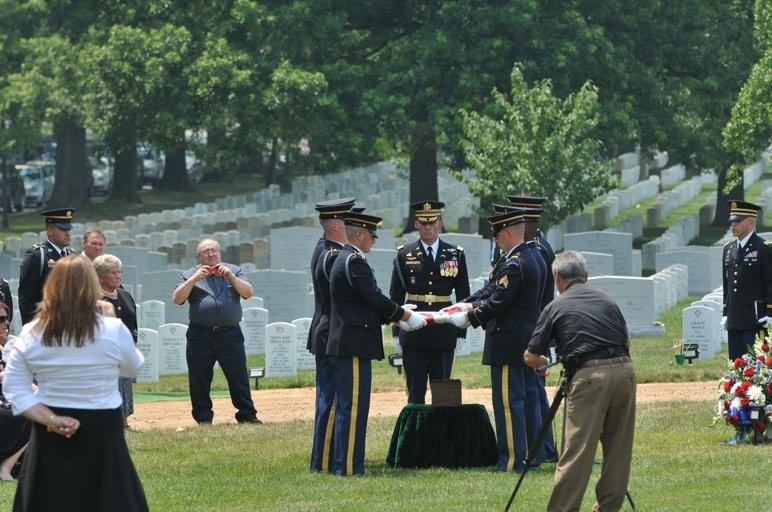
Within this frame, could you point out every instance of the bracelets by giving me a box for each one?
[46,413,57,432]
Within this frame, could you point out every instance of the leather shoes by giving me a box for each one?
[524,457,558,468]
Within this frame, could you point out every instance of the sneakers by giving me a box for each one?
[239,419,261,423]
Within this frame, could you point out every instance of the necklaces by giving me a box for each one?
[99,285,117,295]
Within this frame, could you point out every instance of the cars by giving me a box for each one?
[136,146,167,190]
[1,157,57,212]
[89,155,110,193]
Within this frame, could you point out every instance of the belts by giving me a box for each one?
[407,294,451,302]
[213,326,234,333]
[580,356,632,368]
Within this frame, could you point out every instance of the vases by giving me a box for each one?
[675,355,684,365]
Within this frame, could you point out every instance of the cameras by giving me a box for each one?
[206,267,219,276]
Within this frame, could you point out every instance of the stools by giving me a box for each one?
[391,404,497,466]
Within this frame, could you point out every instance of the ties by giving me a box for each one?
[61,251,65,256]
[736,244,742,258]
[427,247,434,267]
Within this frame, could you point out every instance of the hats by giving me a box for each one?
[410,200,445,224]
[728,200,762,222]
[485,196,546,239]
[41,207,77,231]
[316,198,383,238]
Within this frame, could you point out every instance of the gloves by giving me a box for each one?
[400,305,427,332]
[721,316,727,329]
[758,316,772,329]
[447,304,472,330]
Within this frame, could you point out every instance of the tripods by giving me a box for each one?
[504,357,637,512]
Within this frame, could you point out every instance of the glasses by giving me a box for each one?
[0,315,8,323]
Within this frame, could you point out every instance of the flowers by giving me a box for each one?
[703,328,772,445]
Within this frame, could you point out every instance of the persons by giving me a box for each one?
[92,253,137,432]
[0,277,14,325]
[525,250,636,511]
[390,201,471,405]
[0,302,33,484]
[172,239,264,426]
[2,254,151,512]
[77,229,106,265]
[441,195,558,474]
[16,205,79,330]
[719,198,772,372]
[309,197,427,474]
